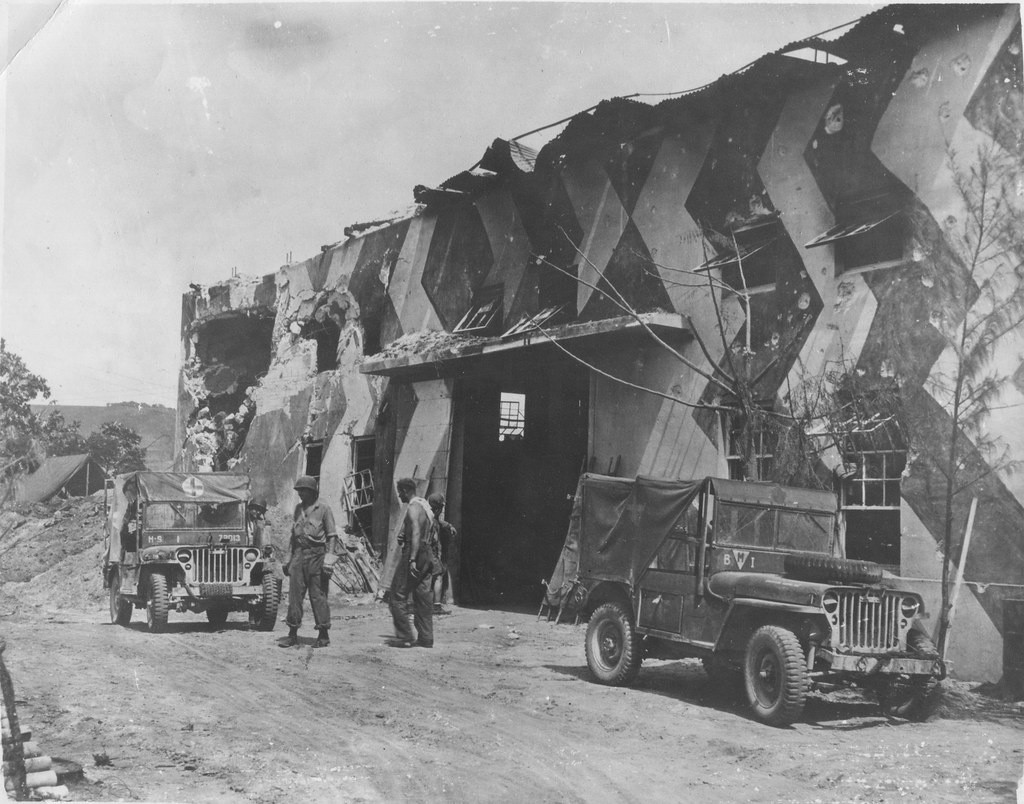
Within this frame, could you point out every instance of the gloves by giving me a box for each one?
[322,553,339,579]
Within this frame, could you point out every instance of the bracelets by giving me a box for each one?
[409,559,416,562]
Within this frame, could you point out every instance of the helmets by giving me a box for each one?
[294,476,317,491]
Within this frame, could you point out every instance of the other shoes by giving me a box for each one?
[433,605,449,614]
[390,639,413,647]
[311,635,330,648]
[416,640,433,647]
[407,603,415,614]
[279,634,297,647]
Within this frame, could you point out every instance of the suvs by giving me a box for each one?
[542,471,947,725]
[105,469,283,630]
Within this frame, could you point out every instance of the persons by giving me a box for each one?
[385,477,440,648]
[278,476,338,647]
[427,494,457,614]
